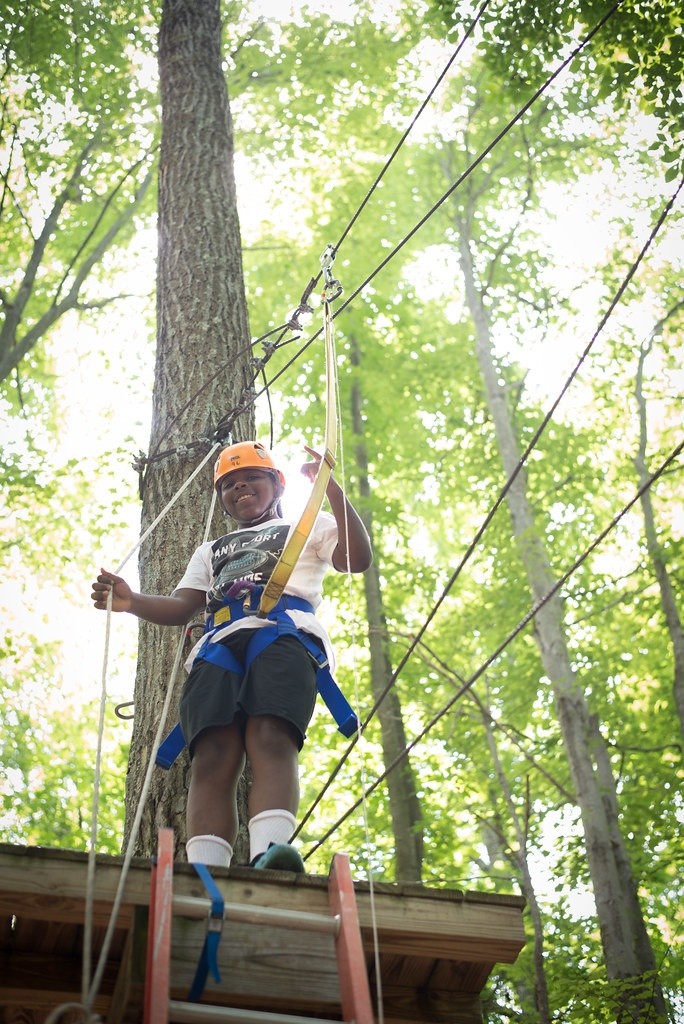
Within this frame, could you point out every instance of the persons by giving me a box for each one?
[89,443,373,874]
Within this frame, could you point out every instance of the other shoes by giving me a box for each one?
[253,844,305,874]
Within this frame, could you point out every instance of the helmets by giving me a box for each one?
[213,441,286,492]
